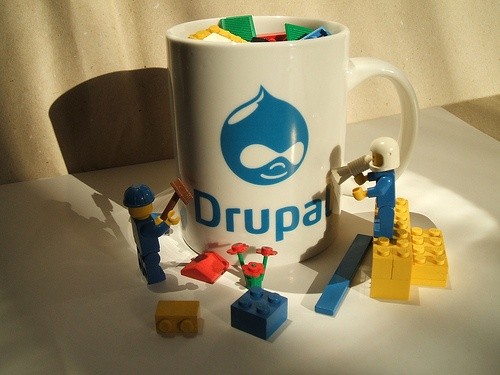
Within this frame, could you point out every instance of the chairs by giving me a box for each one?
[48,66,179,175]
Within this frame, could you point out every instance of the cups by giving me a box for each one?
[167,15,420,267]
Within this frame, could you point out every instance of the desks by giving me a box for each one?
[0,92,500,375]
